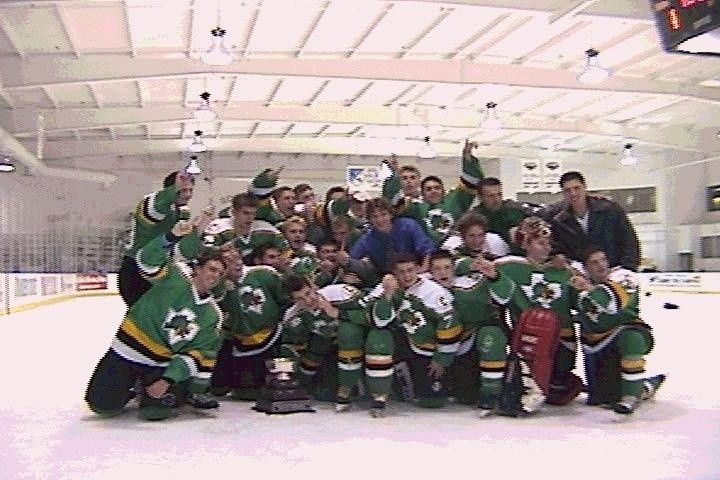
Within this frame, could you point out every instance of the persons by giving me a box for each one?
[83,137,666,422]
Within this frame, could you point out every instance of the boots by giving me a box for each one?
[612,395,641,423]
[648,373,668,403]
[367,395,388,417]
[477,395,495,419]
[334,392,353,414]
[188,395,220,417]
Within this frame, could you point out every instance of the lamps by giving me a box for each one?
[0,157,16,174]
[577,48,610,89]
[200,1,235,66]
[189,129,205,152]
[618,142,639,167]
[186,155,202,175]
[192,77,217,122]
[416,136,437,159]
[480,103,503,129]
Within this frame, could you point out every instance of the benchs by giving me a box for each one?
[0,227,130,273]
